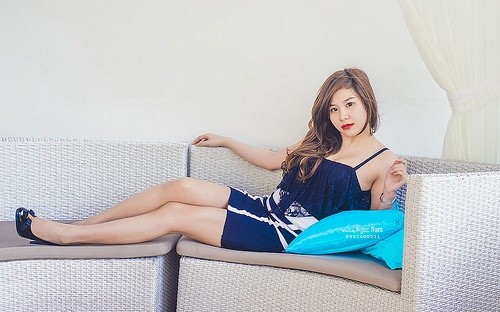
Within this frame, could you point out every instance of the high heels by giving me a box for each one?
[15,207,53,245]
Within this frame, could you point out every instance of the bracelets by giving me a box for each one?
[379,192,396,204]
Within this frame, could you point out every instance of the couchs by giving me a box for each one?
[175,144,500,312]
[0,136,189,312]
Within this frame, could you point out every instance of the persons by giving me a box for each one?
[15,68,409,253]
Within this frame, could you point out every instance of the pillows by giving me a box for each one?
[359,226,405,270]
[281,209,404,254]
